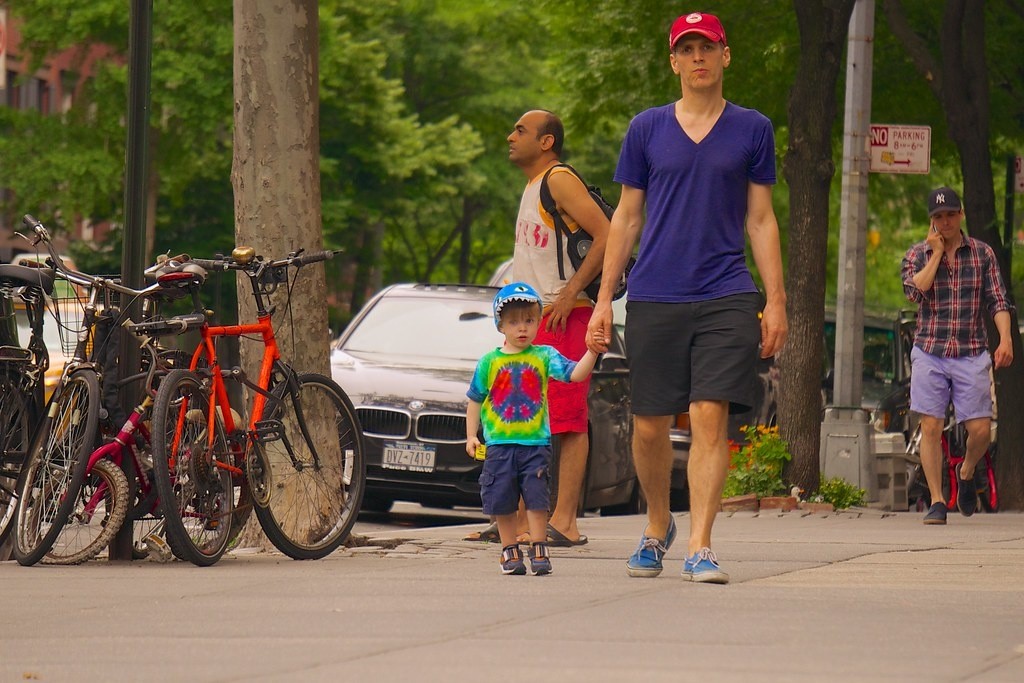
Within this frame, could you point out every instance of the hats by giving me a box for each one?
[928,187,961,218]
[669,12,726,52]
[494,282,542,328]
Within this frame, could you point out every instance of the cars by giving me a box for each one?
[819,309,916,446]
[329,282,647,525]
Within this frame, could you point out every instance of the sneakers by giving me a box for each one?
[681,548,729,583]
[626,512,678,577]
[500,542,553,574]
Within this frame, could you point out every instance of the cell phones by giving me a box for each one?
[933,225,937,233]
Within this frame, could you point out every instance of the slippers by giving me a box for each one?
[462,522,501,543]
[517,523,587,547]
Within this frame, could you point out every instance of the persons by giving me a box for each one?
[462,110,611,547]
[583,12,789,585]
[465,285,605,576]
[901,187,1015,525]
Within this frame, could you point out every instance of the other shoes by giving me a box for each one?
[924,501,947,524]
[956,462,978,517]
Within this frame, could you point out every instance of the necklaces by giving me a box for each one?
[960,239,963,248]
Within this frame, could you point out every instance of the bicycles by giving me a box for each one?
[875,309,999,513]
[128,244,368,568]
[0,213,254,565]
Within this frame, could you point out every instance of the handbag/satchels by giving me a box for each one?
[540,164,636,304]
